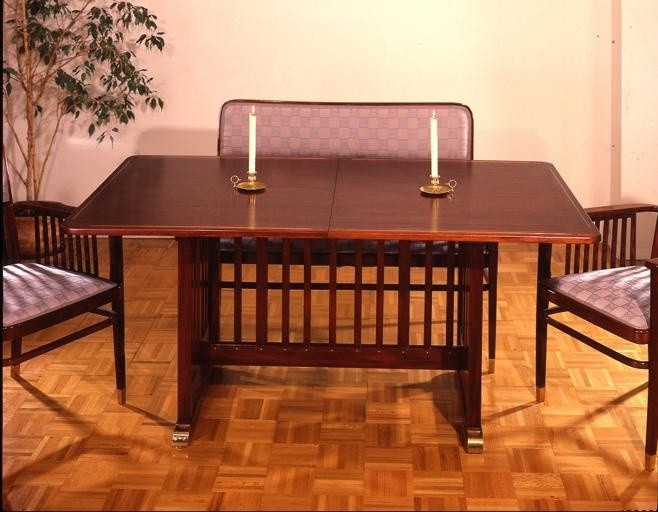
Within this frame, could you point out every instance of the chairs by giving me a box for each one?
[1,201,129,408]
[533,204,658,473]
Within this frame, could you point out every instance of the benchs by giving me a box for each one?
[210,97,500,377]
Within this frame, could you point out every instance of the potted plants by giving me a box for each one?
[0,2,166,269]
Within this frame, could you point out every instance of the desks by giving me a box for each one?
[59,154,602,454]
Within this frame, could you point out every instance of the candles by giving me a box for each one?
[248,115,257,177]
[429,118,439,177]
